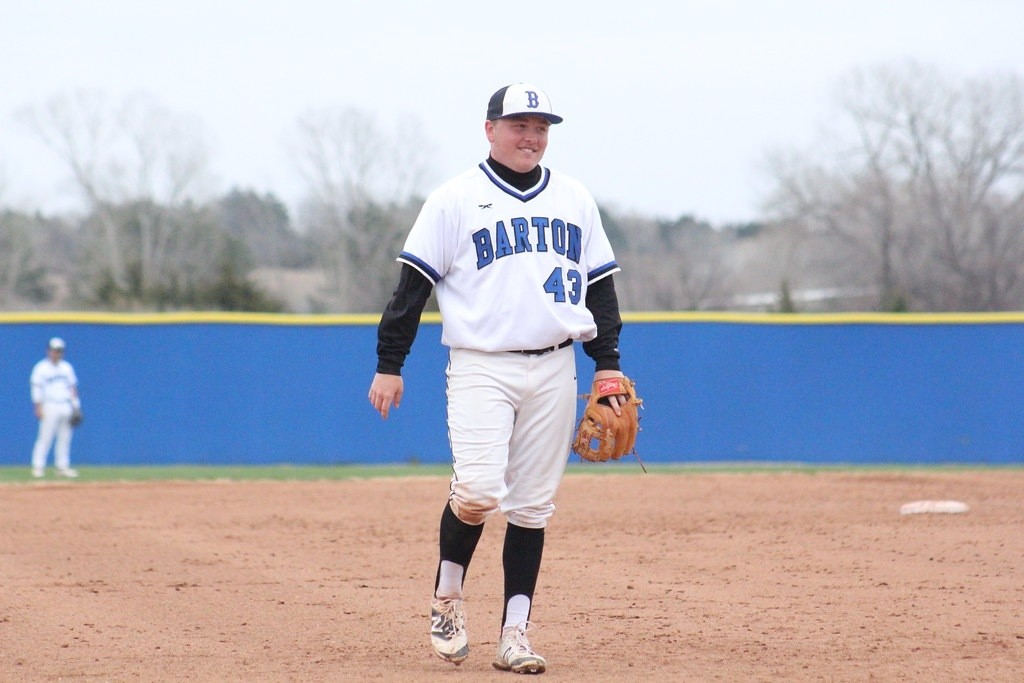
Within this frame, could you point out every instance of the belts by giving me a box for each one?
[507,337,573,355]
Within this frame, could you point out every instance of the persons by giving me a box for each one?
[29,338,82,477]
[368,82,642,674]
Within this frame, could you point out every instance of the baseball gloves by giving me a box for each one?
[69,409,83,428]
[571,375,644,462]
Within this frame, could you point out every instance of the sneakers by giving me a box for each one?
[430,594,469,666]
[492,621,546,674]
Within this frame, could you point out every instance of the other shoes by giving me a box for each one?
[31,469,44,478]
[57,468,78,477]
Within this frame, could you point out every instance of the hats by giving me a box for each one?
[49,337,66,353]
[486,83,563,124]
